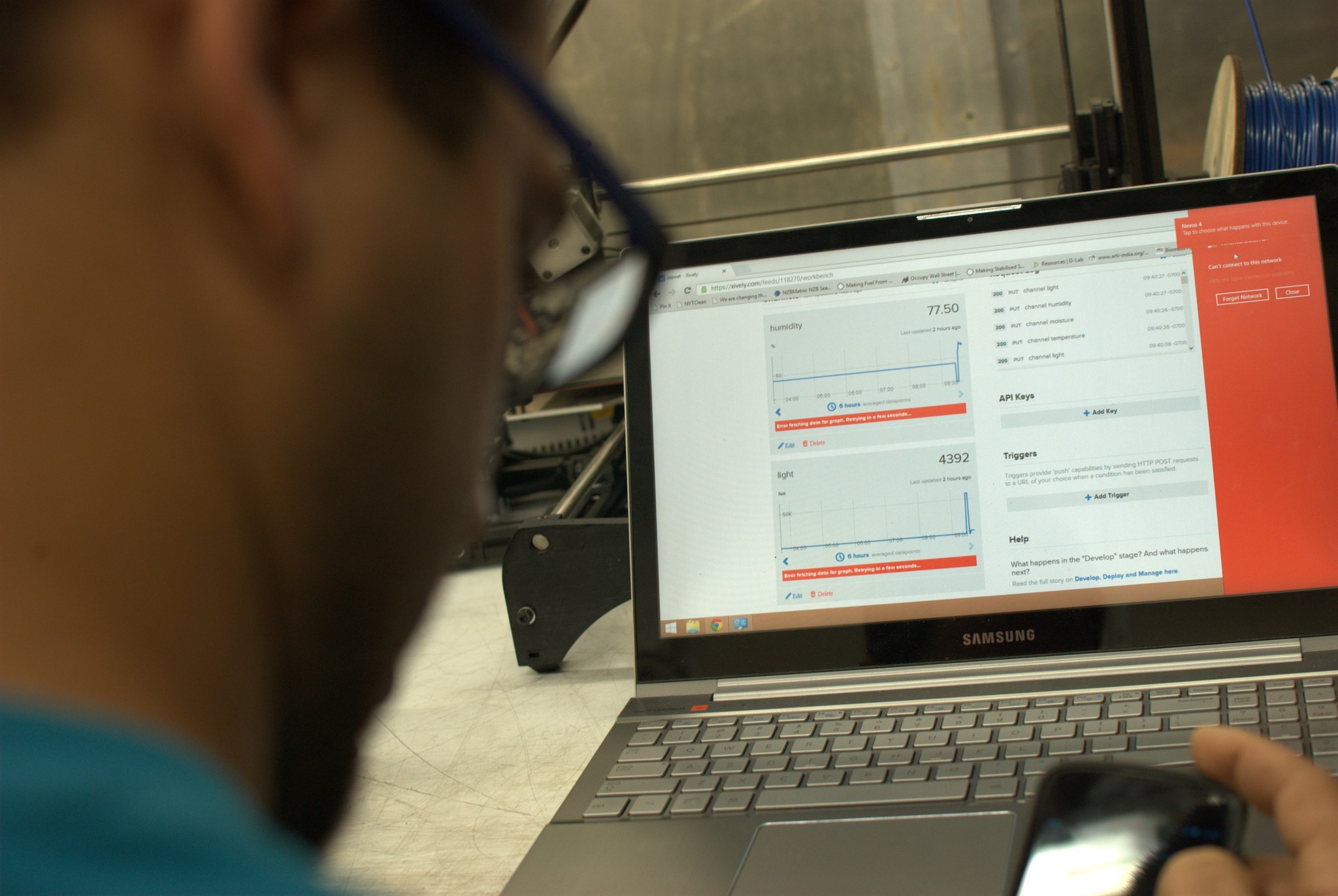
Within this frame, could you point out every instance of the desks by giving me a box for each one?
[323,561,637,896]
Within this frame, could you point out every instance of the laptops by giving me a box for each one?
[497,153,1338,894]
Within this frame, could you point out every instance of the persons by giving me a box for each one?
[0,0,1338,896]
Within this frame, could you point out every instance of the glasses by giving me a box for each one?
[431,10,669,405]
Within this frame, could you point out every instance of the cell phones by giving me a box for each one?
[1010,760,1246,896]
